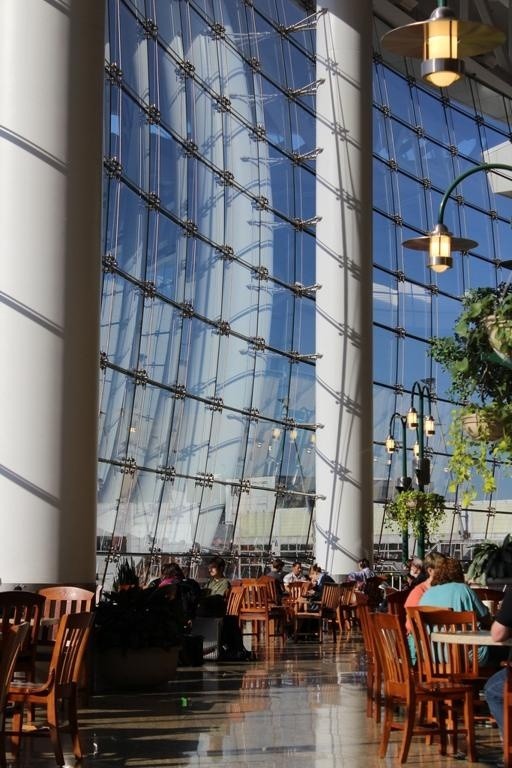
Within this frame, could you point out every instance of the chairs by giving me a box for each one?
[366,577,512,768]
[1,576,94,768]
[225,575,365,689]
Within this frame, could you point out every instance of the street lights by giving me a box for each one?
[386,408,419,566]
[408,379,437,573]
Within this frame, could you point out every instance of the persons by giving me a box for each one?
[294,563,336,644]
[267,558,298,644]
[404,557,429,588]
[419,558,489,668]
[203,555,232,596]
[158,562,185,587]
[482,583,512,767]
[403,551,446,667]
[148,563,168,588]
[345,558,375,589]
[283,562,307,592]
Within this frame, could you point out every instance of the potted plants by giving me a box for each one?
[386,282,512,535]
[85,577,205,690]
